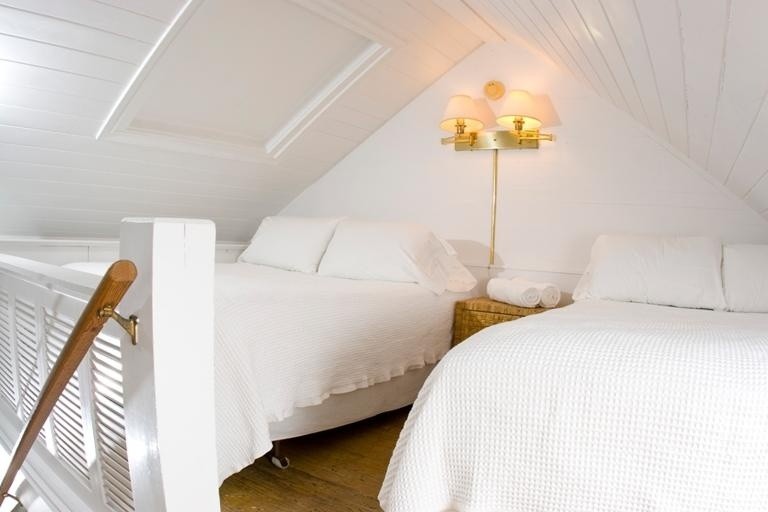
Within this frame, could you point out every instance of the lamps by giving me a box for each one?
[440,90,554,151]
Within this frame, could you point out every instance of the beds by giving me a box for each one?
[385,292,766,512]
[0,206,475,493]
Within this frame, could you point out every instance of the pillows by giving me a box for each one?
[235,211,333,269]
[319,214,454,281]
[568,234,726,303]
[724,223,766,314]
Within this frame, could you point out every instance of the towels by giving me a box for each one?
[485,277,539,308]
[509,277,560,308]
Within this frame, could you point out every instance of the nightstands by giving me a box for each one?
[451,295,557,354]
